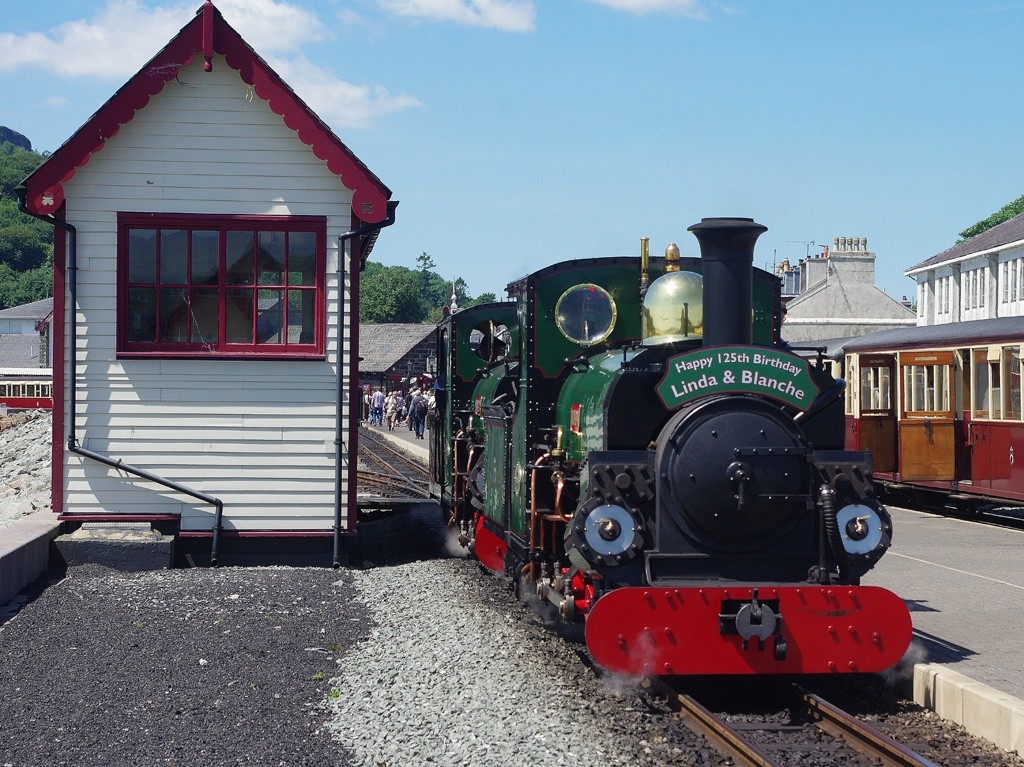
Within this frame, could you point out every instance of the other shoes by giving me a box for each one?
[388,425,394,431]
[415,432,424,439]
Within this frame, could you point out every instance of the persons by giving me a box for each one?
[364,388,385,426]
[384,391,404,431]
[420,388,436,430]
[405,386,428,439]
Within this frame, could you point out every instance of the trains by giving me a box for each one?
[422,216,912,682]
[789,315,1024,515]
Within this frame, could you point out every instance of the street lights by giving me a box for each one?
[406,359,412,392]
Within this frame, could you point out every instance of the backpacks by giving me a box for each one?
[415,397,428,418]
